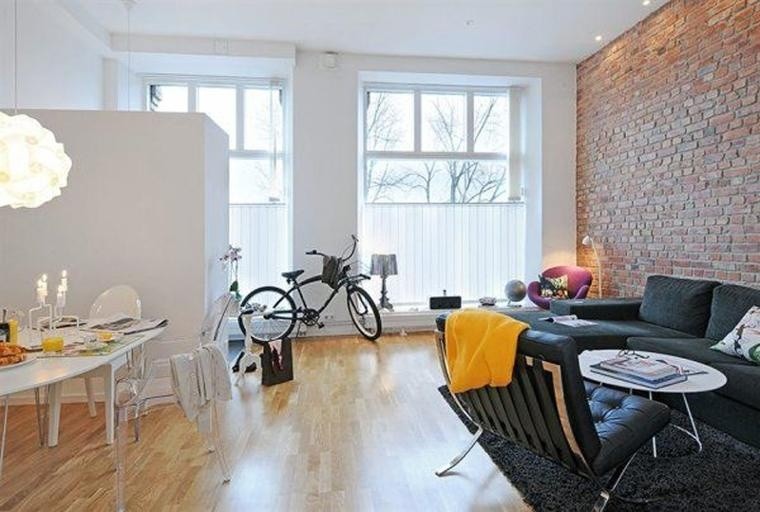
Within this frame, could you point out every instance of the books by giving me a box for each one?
[538,314,597,326]
[590,353,688,389]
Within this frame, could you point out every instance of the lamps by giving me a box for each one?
[370,254,398,312]
[582,234,602,299]
[0,0,72,209]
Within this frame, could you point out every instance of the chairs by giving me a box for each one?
[434,311,672,512]
[528,265,592,310]
[84,284,142,418]
[115,291,235,512]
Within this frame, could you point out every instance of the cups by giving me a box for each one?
[41,329,66,352]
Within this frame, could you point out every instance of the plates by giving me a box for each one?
[0,353,37,370]
[23,334,115,351]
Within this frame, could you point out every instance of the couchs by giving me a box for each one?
[493,275,760,449]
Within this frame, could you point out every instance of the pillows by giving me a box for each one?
[709,304,760,366]
[538,274,568,299]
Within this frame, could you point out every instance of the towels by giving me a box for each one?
[443,308,530,394]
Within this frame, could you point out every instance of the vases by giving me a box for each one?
[228,300,239,313]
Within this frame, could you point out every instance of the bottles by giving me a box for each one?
[0,308,18,343]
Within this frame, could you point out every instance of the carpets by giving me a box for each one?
[437,381,760,512]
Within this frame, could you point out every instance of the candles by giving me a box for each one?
[60,271,69,290]
[37,274,49,302]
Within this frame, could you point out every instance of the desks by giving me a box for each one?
[228,308,274,370]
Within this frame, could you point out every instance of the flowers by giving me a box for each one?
[218,245,242,299]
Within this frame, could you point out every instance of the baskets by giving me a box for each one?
[346,259,370,282]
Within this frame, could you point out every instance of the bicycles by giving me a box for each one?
[238,235,381,343]
